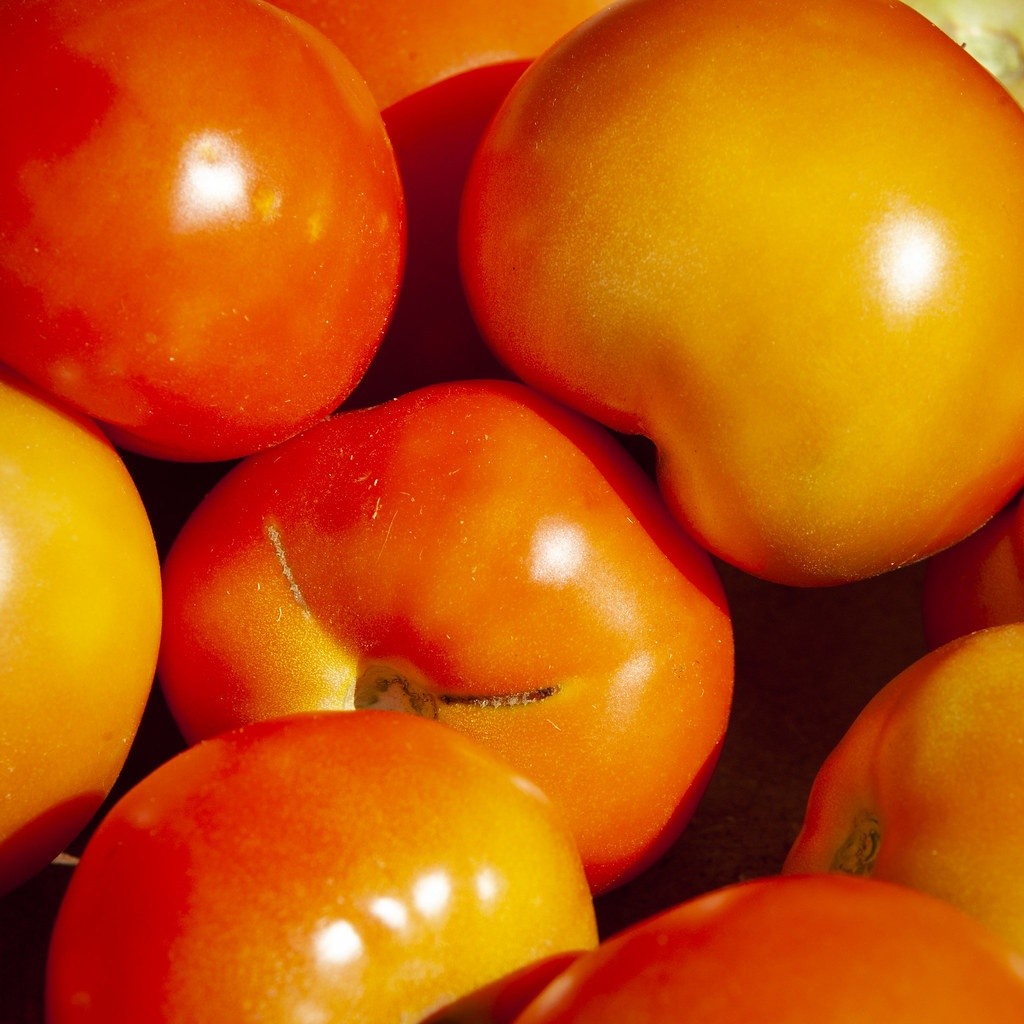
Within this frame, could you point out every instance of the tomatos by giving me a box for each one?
[0,0,1024,1024]
